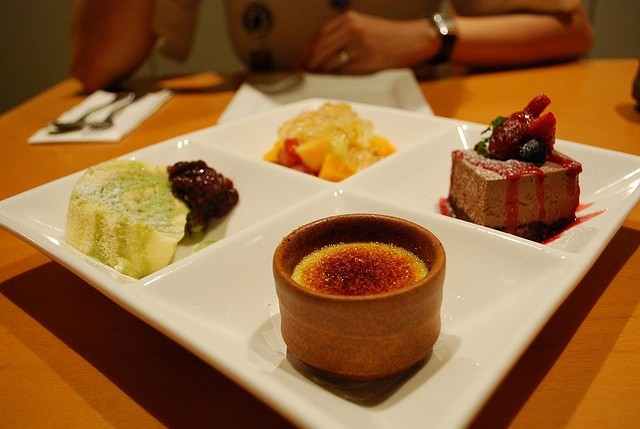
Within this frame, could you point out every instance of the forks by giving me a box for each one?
[87,89,147,129]
[52,94,120,132]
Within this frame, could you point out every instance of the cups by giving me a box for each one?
[272,212,447,382]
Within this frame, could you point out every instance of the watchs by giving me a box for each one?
[430,12,458,64]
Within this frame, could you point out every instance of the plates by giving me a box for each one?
[0,95,640,429]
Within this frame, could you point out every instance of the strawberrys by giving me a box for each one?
[473,110,535,163]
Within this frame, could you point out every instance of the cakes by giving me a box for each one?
[438,93,608,246]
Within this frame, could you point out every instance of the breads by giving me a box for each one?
[63,157,193,281]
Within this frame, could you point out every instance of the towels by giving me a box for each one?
[217,70,433,131]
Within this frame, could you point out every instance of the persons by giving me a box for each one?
[67,1,594,94]
